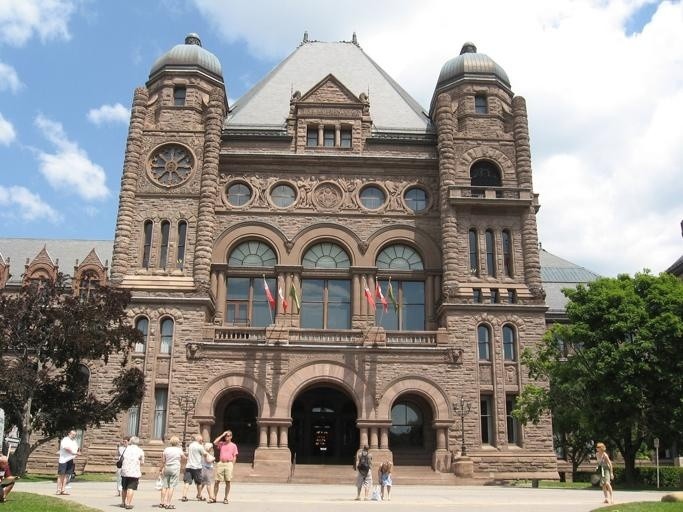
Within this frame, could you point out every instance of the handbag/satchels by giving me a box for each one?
[155,473,165,491]
[116,460,122,468]
[372,485,381,501]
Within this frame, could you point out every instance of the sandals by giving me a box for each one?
[119,496,230,510]
[55,490,71,495]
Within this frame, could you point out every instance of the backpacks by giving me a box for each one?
[356,451,370,472]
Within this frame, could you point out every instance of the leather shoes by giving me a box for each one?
[354,497,391,502]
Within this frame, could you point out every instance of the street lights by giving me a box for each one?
[452,398,471,456]
[178,392,197,451]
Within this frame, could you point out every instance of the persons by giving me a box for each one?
[181,436,214,501]
[196,442,215,499]
[207,430,238,503]
[54,429,81,494]
[116,436,144,509]
[115,437,129,496]
[153,435,188,508]
[377,459,391,501]
[353,445,372,500]
[0,453,14,502]
[594,442,614,503]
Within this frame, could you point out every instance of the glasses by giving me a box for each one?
[596,446,601,449]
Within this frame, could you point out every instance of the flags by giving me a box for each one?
[264,280,275,310]
[375,281,386,312]
[387,281,397,314]
[363,285,376,312]
[288,282,301,314]
[278,285,287,310]
